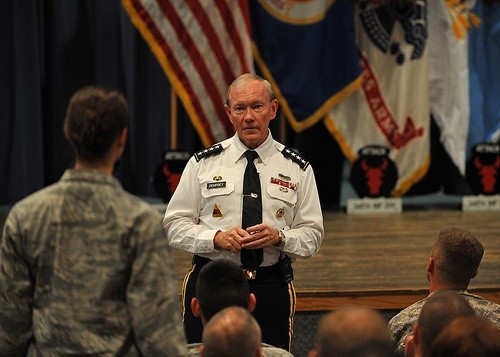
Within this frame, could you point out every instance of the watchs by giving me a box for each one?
[271,228,285,247]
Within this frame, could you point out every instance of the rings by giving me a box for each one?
[260,239,263,243]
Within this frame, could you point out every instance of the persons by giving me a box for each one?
[185,259,294,357]
[0,86,190,357]
[163,73,325,353]
[388,226,500,357]
[308,305,395,357]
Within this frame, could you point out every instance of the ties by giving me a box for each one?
[241,150,264,272]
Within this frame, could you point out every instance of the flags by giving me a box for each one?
[122,0,500,197]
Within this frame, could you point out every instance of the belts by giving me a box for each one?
[192,255,292,282]
